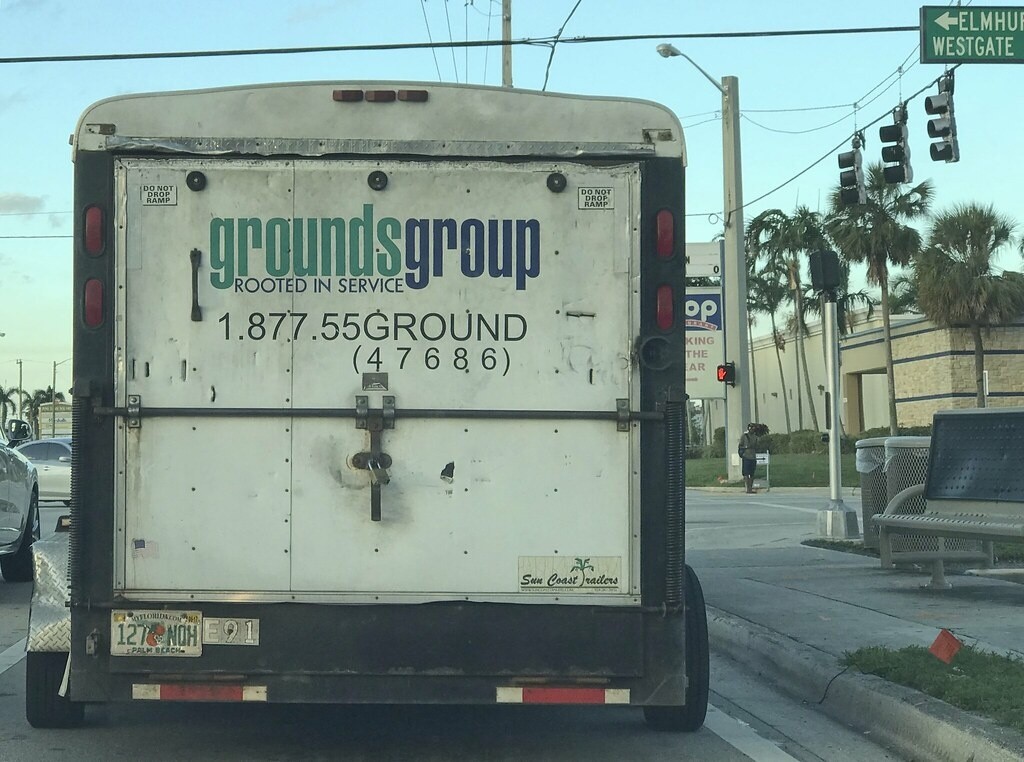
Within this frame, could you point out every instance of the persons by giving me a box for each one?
[738,423,759,494]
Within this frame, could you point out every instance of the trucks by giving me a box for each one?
[23,75,712,732]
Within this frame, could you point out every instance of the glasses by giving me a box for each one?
[747,425,757,431]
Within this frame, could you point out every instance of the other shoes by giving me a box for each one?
[745,490,757,495]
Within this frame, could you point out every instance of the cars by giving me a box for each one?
[0,416,41,580]
[11,437,73,506]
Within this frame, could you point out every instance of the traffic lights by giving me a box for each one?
[717,362,735,384]
[925,94,959,162]
[836,148,868,205]
[879,122,911,186]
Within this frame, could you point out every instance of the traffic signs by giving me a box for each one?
[920,5,1024,64]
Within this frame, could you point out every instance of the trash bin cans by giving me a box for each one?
[854,436,890,550]
[884,436,968,553]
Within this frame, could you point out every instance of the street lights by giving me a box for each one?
[658,39,751,484]
[809,250,861,540]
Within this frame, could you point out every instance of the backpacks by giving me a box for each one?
[738,444,746,458]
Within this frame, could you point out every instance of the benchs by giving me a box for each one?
[870,406,1024,590]
